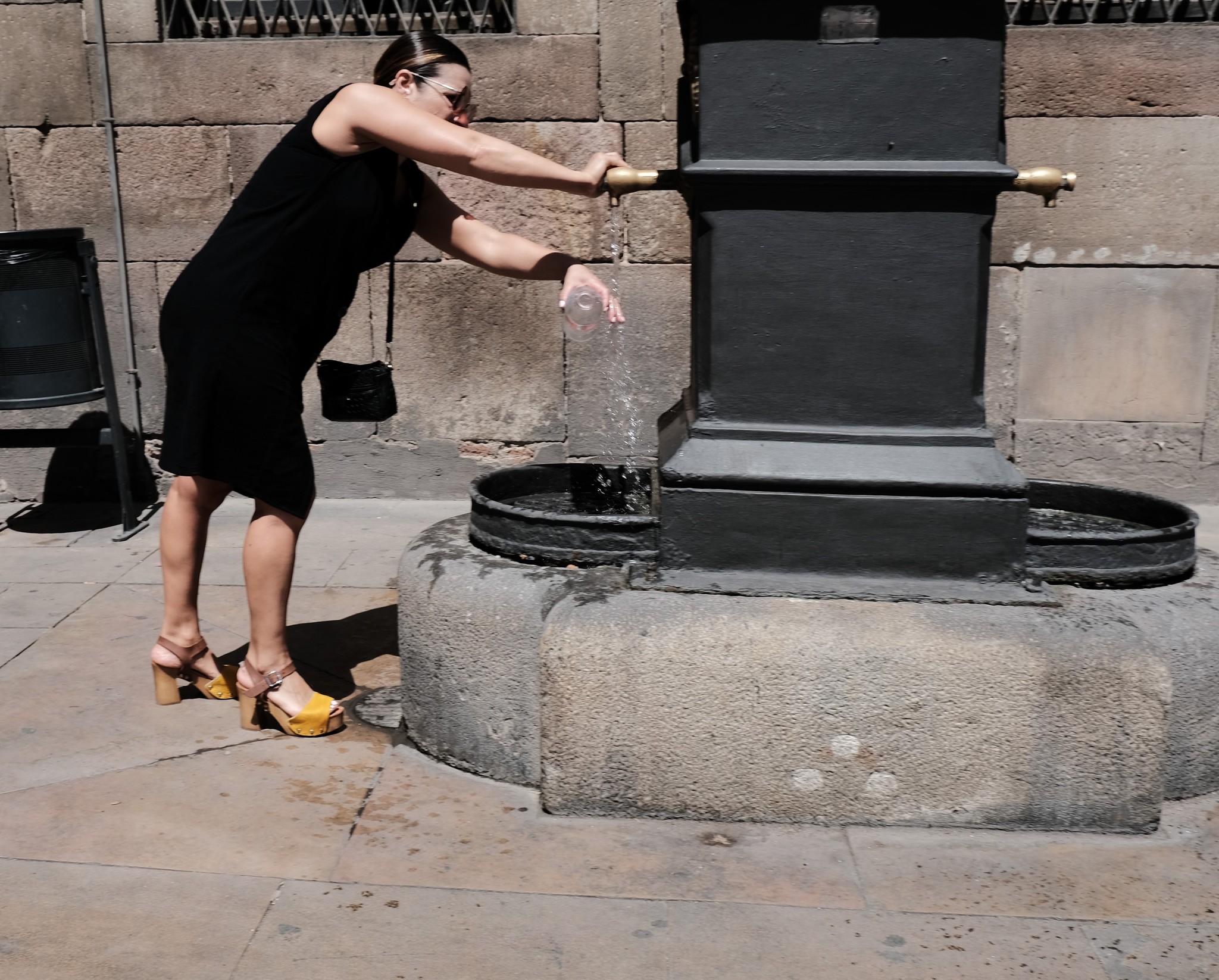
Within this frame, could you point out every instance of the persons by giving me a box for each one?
[150,30,629,740]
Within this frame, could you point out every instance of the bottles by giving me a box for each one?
[563,285,605,343]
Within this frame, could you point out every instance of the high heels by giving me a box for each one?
[235,655,346,736]
[150,636,239,706]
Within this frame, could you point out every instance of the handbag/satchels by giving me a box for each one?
[315,360,397,422]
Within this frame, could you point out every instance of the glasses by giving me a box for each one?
[389,71,478,123]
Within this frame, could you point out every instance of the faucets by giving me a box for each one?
[599,165,659,208]
[1003,165,1077,210]
